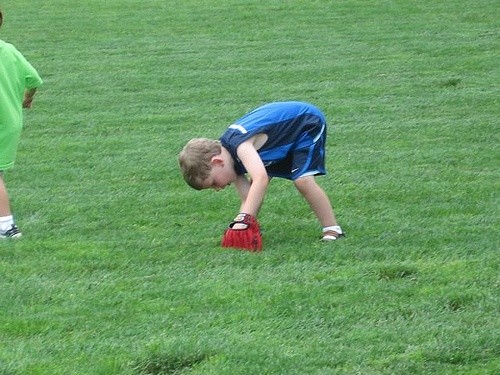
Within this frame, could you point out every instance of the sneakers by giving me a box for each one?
[0,224,22,239]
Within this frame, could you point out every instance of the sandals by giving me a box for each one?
[323,231,344,241]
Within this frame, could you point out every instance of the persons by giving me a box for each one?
[0,10,43,241]
[179,101,345,250]
